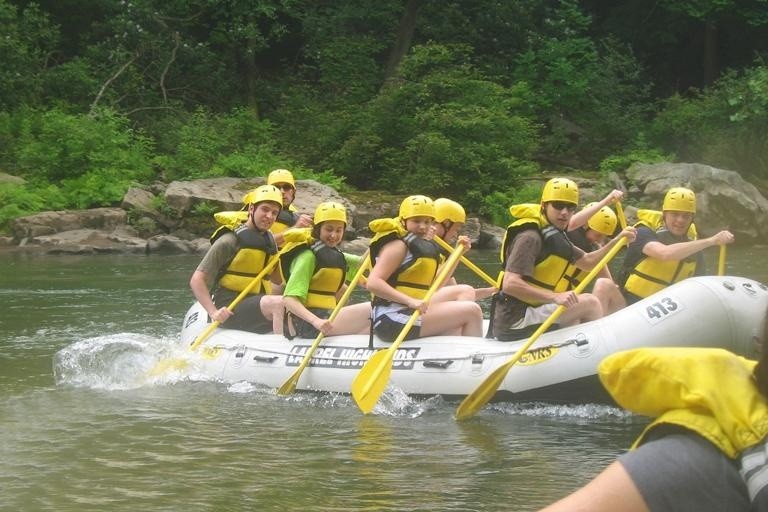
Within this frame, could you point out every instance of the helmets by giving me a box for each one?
[544,179,580,204]
[433,198,468,225]
[250,186,284,206]
[312,202,348,226]
[585,204,618,237]
[269,168,295,189]
[396,197,437,222]
[663,186,696,213]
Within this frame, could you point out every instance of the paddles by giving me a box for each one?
[148,244,290,376]
[456,236,629,422]
[277,253,370,396]
[351,242,465,415]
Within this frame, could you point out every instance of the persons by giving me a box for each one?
[616,188,736,308]
[491,177,635,341]
[432,198,496,302]
[283,204,371,338]
[194,185,296,340]
[266,169,313,253]
[566,189,626,288]
[367,194,483,343]
[539,310,767,512]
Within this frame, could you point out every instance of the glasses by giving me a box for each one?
[551,201,578,211]
[274,185,294,190]
